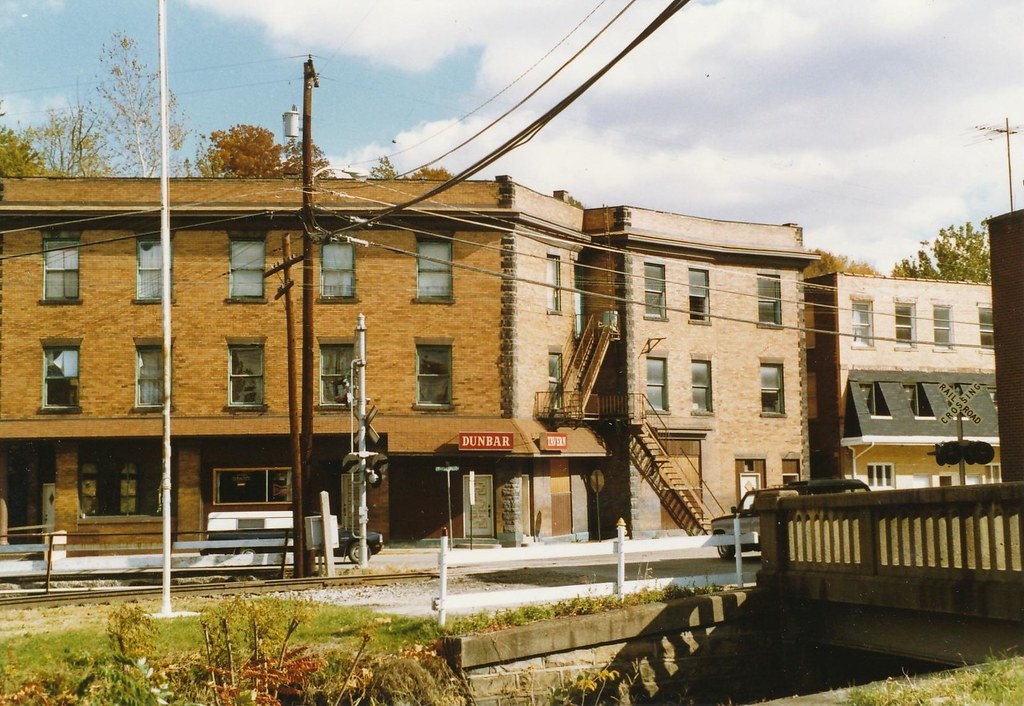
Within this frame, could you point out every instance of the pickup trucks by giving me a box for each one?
[200,528,383,565]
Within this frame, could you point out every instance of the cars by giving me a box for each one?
[710,479,871,559]
